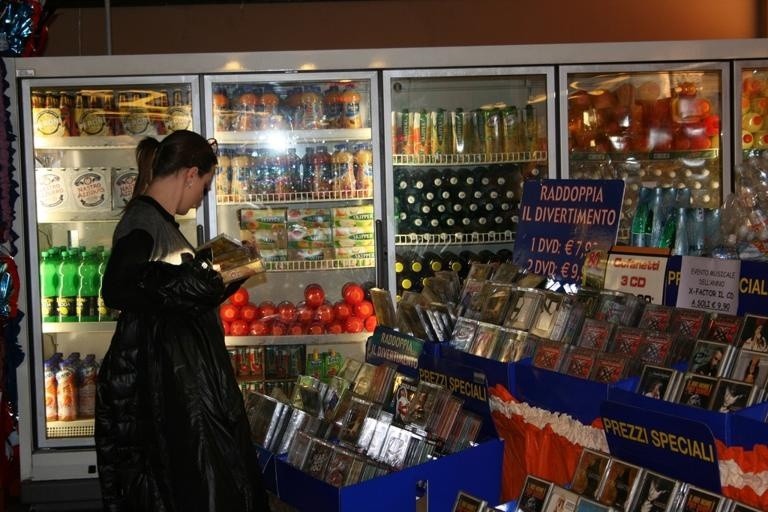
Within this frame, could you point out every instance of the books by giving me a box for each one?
[368,260,768,415]
[194,232,264,283]
[242,359,483,489]
[452,449,758,512]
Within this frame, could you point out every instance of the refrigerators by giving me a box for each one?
[1,39,768,484]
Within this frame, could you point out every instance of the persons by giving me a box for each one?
[97,128,268,511]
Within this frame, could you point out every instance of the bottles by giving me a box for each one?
[213,83,363,133]
[30,81,193,136]
[44,350,107,424]
[213,141,371,198]
[566,79,768,260]
[393,162,549,300]
[40,246,122,325]
[219,280,382,334]
